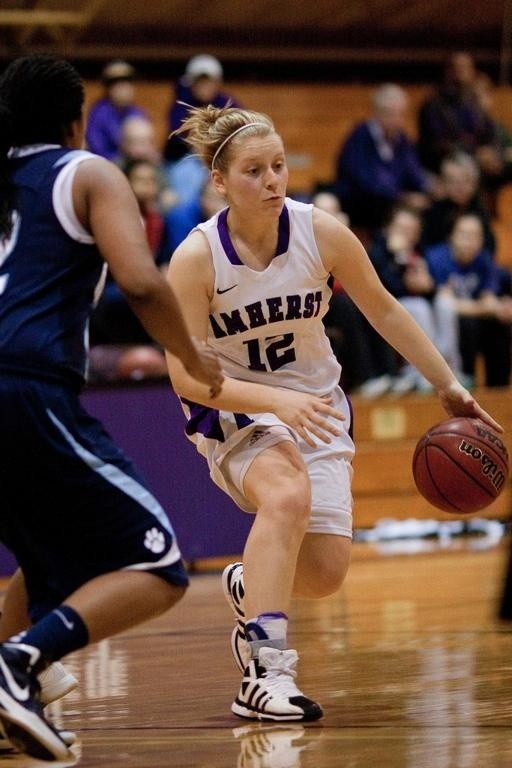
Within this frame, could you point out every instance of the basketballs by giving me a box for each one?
[413,417,508,513]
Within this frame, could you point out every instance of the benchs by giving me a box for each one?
[75,78,512,537]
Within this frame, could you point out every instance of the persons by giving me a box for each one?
[165,100,505,722]
[85,53,236,385]
[0,57,225,768]
[309,53,509,394]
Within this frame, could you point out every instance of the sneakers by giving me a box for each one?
[222,561,252,675]
[231,646,323,721]
[0,642,72,762]
[0,720,77,752]
[36,659,80,705]
